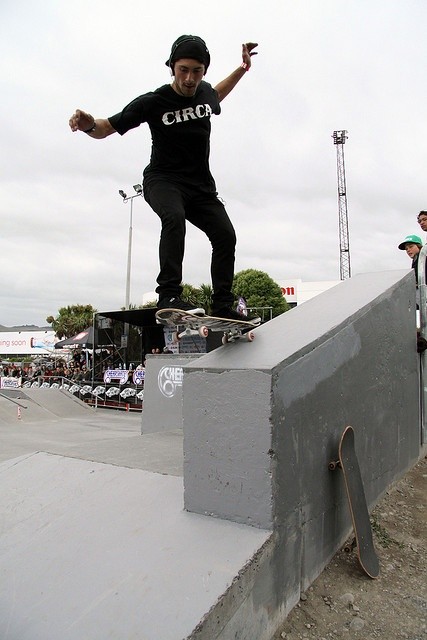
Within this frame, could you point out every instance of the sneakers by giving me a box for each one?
[155,295,205,324]
[210,301,261,323]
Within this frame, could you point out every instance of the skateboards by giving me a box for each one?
[329,425,380,579]
[155,309,260,344]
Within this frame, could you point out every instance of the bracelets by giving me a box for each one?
[240,63,249,71]
[83,123,95,133]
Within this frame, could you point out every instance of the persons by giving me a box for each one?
[398,235,427,310]
[416,211,426,231]
[68,35,262,326]
[0,344,114,381]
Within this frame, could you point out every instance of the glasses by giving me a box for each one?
[418,217,427,223]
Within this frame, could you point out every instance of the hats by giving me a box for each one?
[166,36,210,76]
[398,235,422,250]
